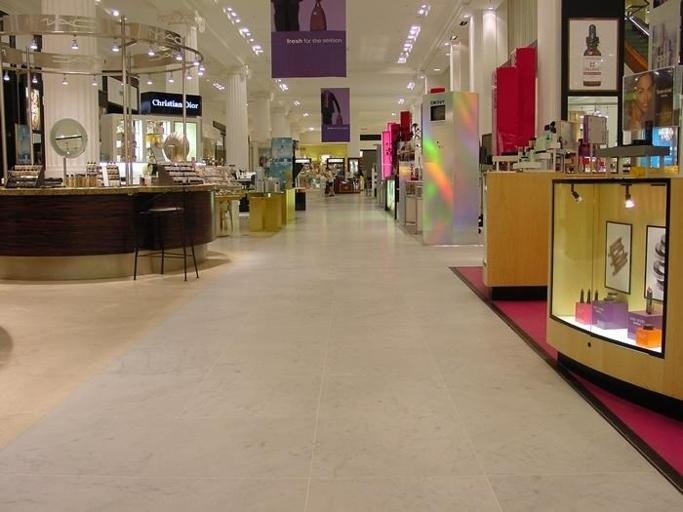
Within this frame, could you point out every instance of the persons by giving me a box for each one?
[30,88,42,131]
[320,88,342,124]
[322,167,333,195]
[254,162,265,183]
[624,70,656,130]
[269,0,305,32]
[354,169,364,192]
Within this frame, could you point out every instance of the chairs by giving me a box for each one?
[130,187,197,281]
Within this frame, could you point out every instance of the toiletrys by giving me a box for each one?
[64,161,120,190]
[508,120,586,175]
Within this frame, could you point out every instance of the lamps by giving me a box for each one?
[570,184,583,205]
[621,183,634,209]
